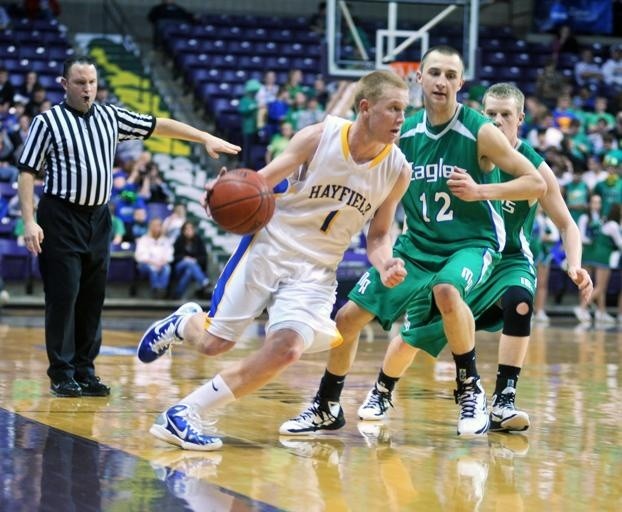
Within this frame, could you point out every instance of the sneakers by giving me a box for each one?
[453,376,489,438]
[81,377,111,397]
[574,306,591,322]
[488,387,530,432]
[595,310,616,323]
[279,400,346,435]
[535,311,549,322]
[149,405,223,451]
[137,302,203,363]
[358,383,392,420]
[49,378,82,397]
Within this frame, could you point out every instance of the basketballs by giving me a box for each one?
[208,168,277,237]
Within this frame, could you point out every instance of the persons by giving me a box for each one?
[534,205,561,322]
[578,194,602,274]
[358,82,594,433]
[172,221,216,298]
[0,69,51,248]
[111,151,145,247]
[518,25,622,215]
[18,54,241,398]
[144,153,174,206]
[138,71,413,452]
[574,203,622,323]
[240,68,330,163]
[164,202,188,244]
[280,44,548,439]
[135,218,172,298]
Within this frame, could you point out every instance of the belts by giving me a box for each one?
[46,193,103,211]
